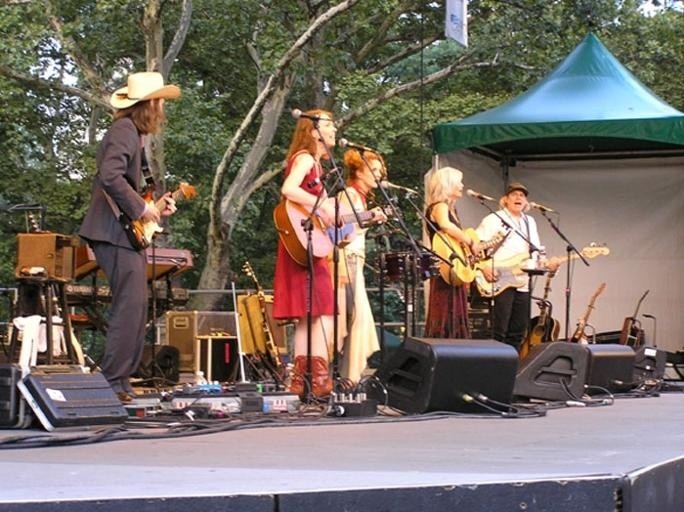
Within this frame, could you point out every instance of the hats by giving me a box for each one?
[110,72,180,109]
[506,181,528,196]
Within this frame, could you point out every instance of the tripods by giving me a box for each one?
[135,237,170,390]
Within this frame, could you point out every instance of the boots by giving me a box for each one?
[290,356,333,397]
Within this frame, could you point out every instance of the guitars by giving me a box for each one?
[473,242,610,300]
[272,195,392,268]
[620,290,649,348]
[432,226,503,288]
[568,282,607,346]
[123,178,197,250]
[241,262,283,367]
[519,267,561,359]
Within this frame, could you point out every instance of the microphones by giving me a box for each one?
[336,135,379,156]
[290,108,334,121]
[467,188,498,202]
[529,201,556,213]
[306,166,342,188]
[380,179,419,195]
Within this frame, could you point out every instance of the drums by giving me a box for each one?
[385,250,440,284]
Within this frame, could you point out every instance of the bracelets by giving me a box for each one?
[469,239,473,247]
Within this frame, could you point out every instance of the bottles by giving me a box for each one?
[193,370,208,387]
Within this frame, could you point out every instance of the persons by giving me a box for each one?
[475,182,560,351]
[425,166,481,339]
[272,109,348,395]
[78,72,182,404]
[334,149,386,388]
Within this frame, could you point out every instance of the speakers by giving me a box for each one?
[372,336,521,413]
[514,340,590,403]
[634,345,670,386]
[141,342,181,386]
[585,344,636,399]
